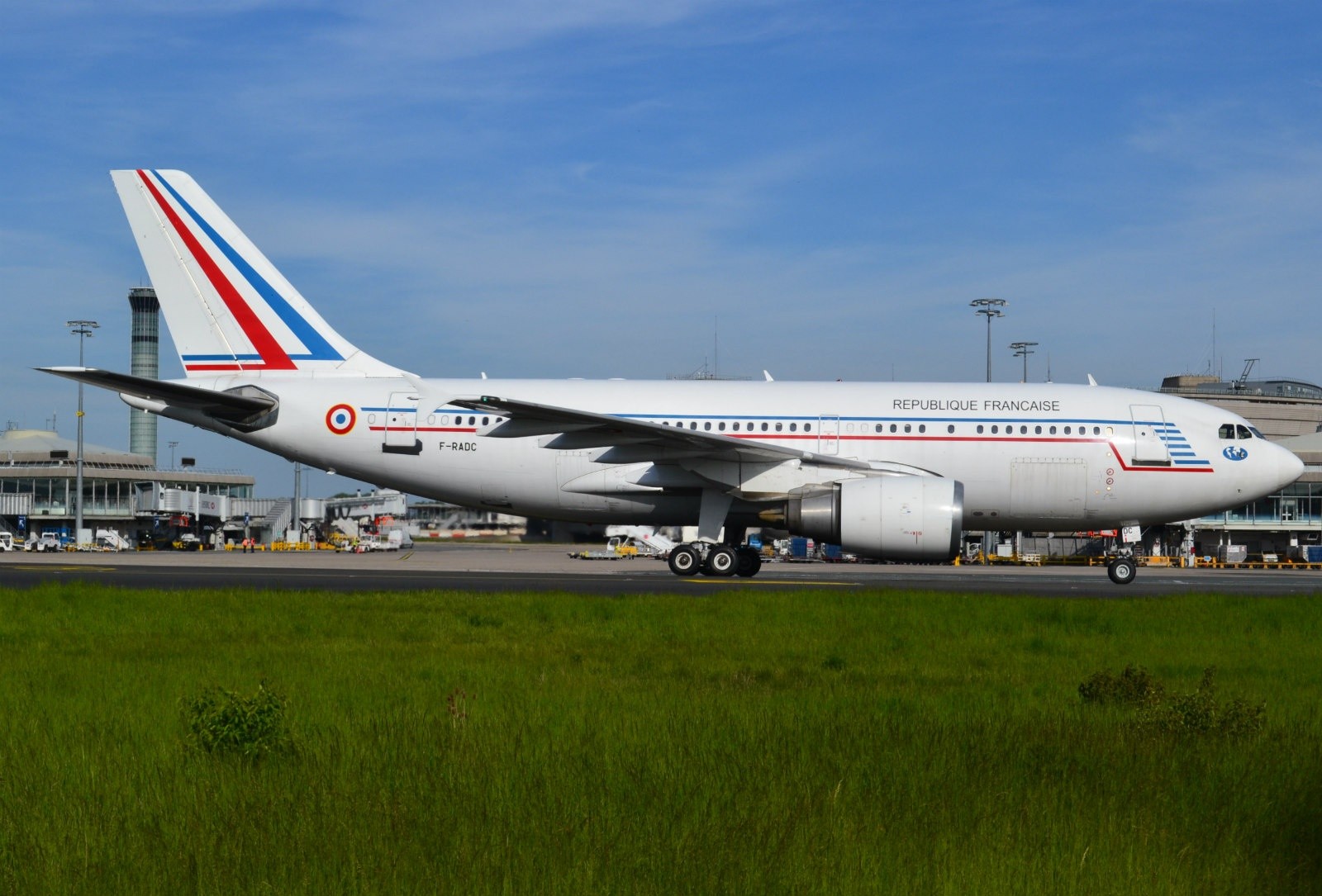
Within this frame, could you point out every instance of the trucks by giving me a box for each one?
[25,532,61,554]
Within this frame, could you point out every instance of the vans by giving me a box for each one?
[387,531,414,549]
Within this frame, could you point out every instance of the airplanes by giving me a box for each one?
[28,170,1305,583]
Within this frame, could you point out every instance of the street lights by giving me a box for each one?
[64,320,100,543]
[969,299,1010,566]
[1010,342,1039,566]
[167,441,180,472]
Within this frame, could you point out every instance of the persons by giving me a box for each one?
[242,537,249,553]
[250,537,255,553]
